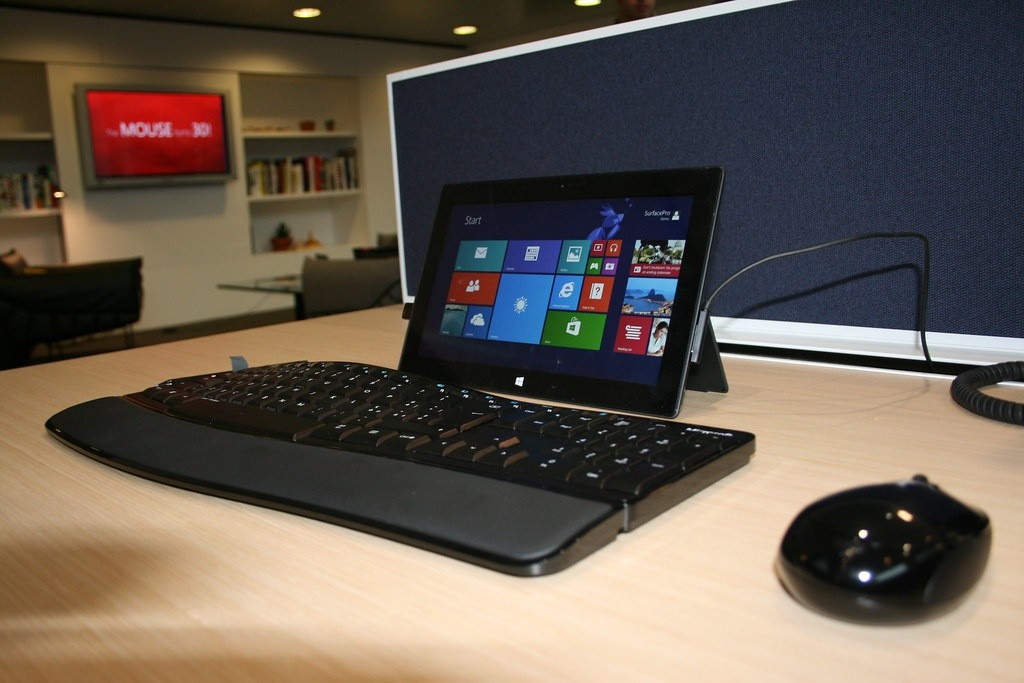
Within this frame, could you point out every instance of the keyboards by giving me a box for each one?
[44,359,757,577]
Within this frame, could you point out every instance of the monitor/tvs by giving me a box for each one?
[73,81,238,187]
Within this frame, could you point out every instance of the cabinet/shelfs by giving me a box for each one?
[237,69,370,255]
[0,58,70,263]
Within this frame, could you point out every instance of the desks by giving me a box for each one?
[217,274,303,293]
[0,304,1024,683]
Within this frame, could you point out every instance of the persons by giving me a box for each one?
[647,321,669,357]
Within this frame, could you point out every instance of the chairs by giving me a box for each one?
[0,247,144,370]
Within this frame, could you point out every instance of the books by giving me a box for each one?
[0,164,59,212]
[247,146,360,196]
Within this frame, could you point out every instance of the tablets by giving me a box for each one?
[399,166,725,419]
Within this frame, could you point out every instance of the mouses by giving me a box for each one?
[774,474,993,627]
